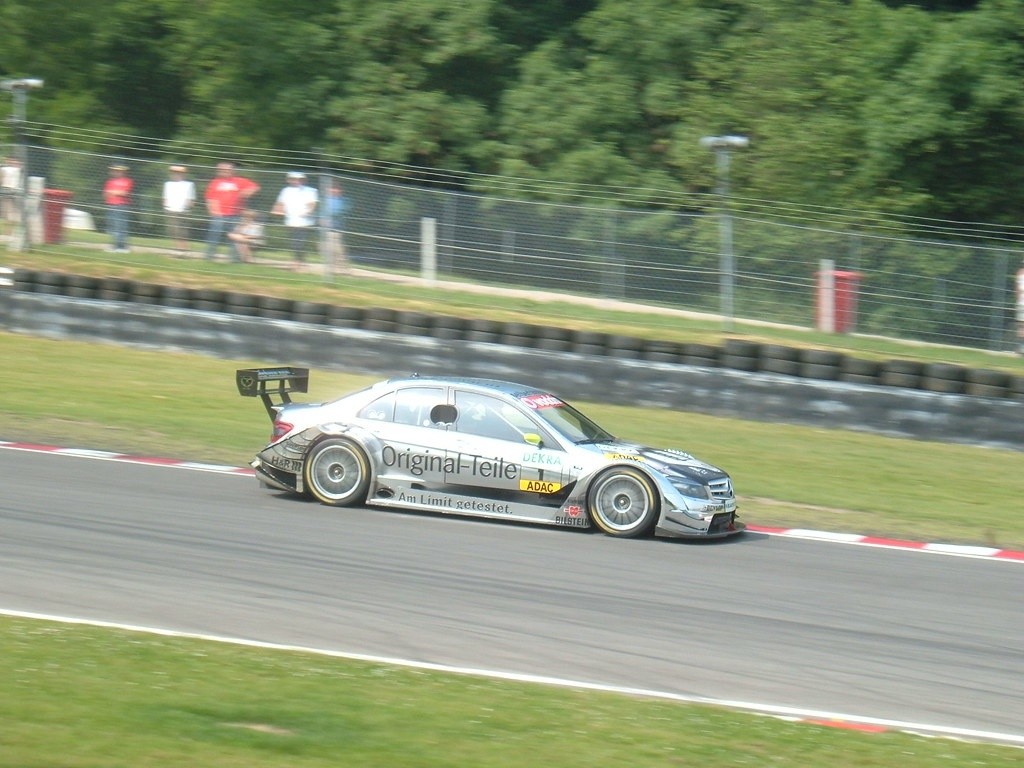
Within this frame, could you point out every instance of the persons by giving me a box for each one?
[163,165,197,257]
[103,164,134,252]
[0,154,28,252]
[304,184,356,272]
[488,399,509,431]
[204,162,266,264]
[1013,268,1024,355]
[270,172,320,271]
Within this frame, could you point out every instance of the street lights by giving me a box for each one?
[700,135,754,320]
[2,76,43,164]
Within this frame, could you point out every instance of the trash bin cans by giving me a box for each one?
[815,270,865,333]
[45,188,73,244]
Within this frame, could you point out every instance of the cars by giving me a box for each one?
[236,365,742,539]
